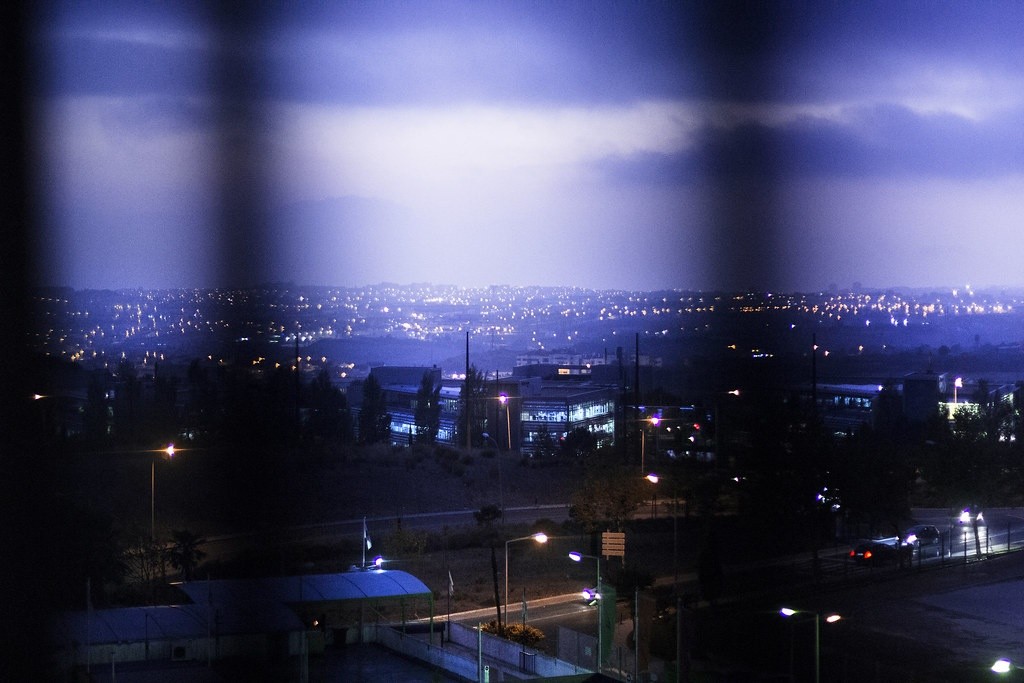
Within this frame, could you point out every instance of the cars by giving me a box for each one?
[851,536,913,567]
[905,525,940,548]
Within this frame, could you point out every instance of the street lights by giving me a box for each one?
[501,528,547,628]
[636,410,659,476]
[496,392,512,450]
[952,376,963,405]
[567,549,604,679]
[145,444,177,545]
